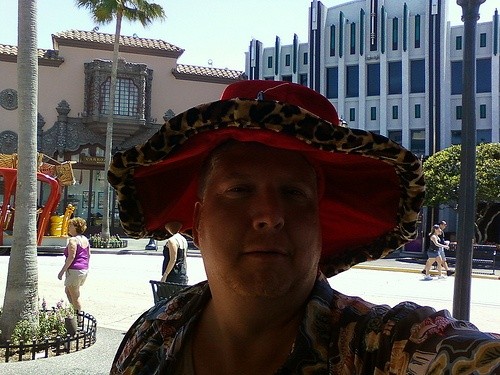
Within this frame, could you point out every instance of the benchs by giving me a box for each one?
[434,244,497,274]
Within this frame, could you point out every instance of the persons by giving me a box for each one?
[107,79,499,374]
[422,220,455,280]
[58,217,90,326]
[160,221,188,285]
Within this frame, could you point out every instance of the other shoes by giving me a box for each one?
[447,270,455,276]
[438,275,445,279]
[422,270,426,274]
[425,274,433,280]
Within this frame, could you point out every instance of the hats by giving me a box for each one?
[107,80,426,278]
